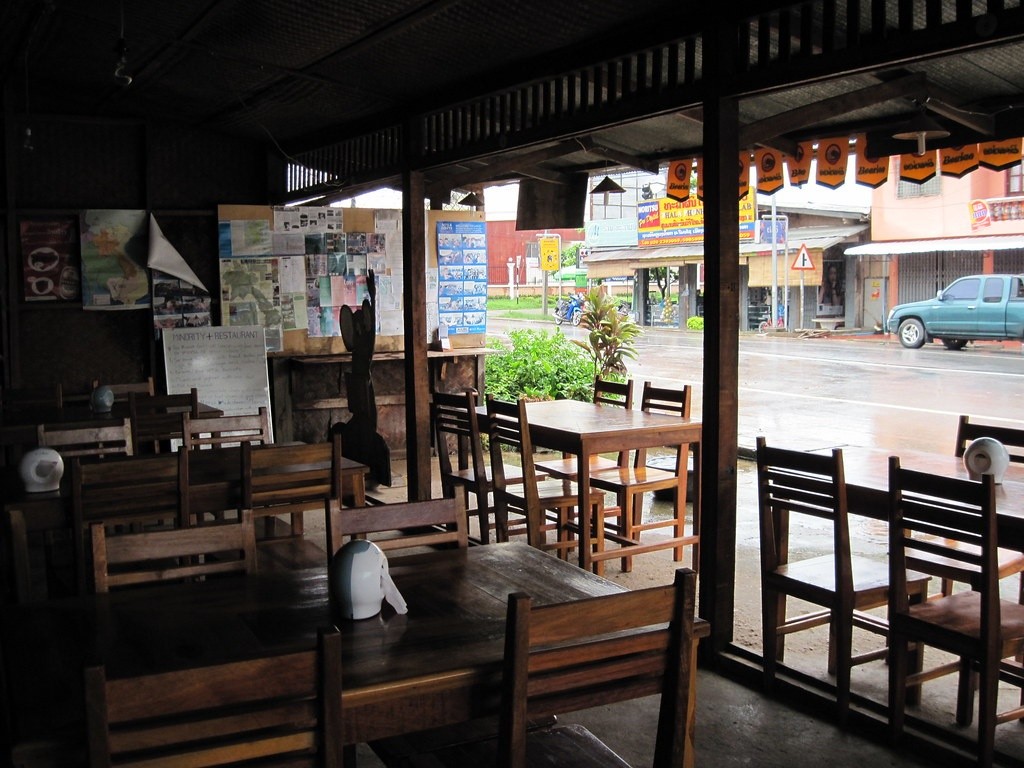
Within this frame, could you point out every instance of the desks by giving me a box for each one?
[456,399,702,571]
[768,443,1024,664]
[0,541,711,768]
[0,403,223,465]
[812,318,845,330]
[0,442,370,603]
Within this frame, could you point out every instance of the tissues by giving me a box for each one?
[21,448,64,492]
[88,386,114,414]
[964,435,1011,483]
[328,539,407,620]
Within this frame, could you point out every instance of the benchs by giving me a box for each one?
[794,327,861,335]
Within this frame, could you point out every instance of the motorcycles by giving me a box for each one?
[552,293,583,326]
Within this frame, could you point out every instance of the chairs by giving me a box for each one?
[128,388,200,456]
[36,417,134,597]
[0,382,63,467]
[400,568,696,768]
[93,376,160,458]
[534,375,633,552]
[888,456,1024,768]
[80,625,343,768]
[324,484,556,768]
[432,390,549,544]
[904,415,1024,602]
[486,393,606,577]
[182,407,307,541]
[90,508,257,593]
[52,446,190,597]
[589,382,691,571]
[204,434,341,582]
[756,435,932,727]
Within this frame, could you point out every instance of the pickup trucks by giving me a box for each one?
[887,273,1024,350]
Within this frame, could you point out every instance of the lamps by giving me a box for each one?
[458,191,484,216]
[589,176,626,206]
